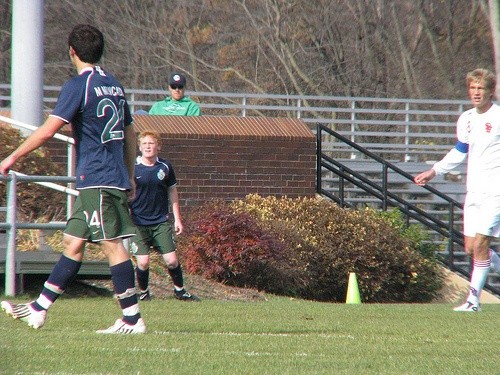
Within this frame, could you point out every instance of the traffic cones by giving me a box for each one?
[344,272,362,304]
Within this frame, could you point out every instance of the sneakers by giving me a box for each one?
[1,300,47,330]
[453,302,479,312]
[174,289,200,301]
[139,291,150,301]
[96,318,147,335]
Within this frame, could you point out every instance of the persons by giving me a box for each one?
[414,68,500,311]
[149,73,201,116]
[0,23,146,334]
[128,130,196,301]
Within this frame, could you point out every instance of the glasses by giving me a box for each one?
[170,84,183,89]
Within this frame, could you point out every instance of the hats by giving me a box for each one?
[169,73,185,87]
